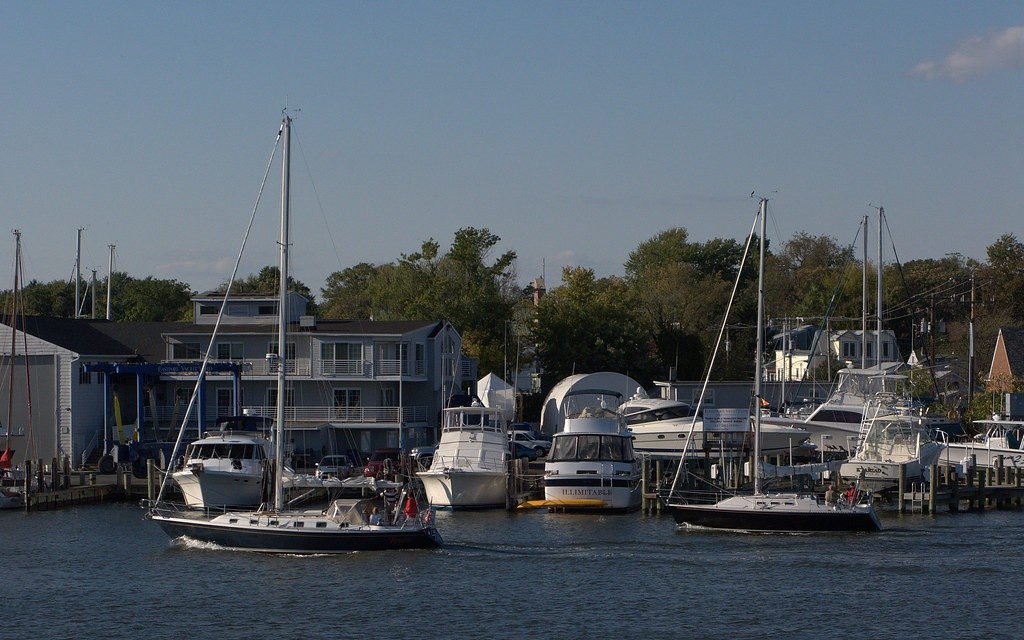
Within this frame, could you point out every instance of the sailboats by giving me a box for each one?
[653,191,884,534]
[138,91,444,555]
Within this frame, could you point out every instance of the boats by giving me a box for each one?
[169,408,274,511]
[412,190,1024,516]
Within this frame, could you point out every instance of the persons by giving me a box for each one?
[847,482,855,504]
[370,506,382,524]
[825,485,838,506]
[404,493,417,526]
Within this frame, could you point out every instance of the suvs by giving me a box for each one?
[507,429,552,458]
[509,422,533,431]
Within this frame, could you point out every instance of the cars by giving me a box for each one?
[507,441,537,463]
[314,454,352,482]
[365,448,399,478]
[408,442,441,468]
